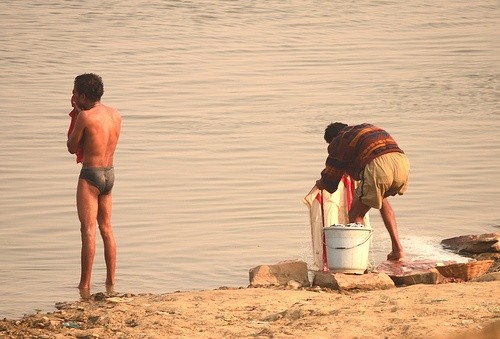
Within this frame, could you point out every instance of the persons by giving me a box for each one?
[67,74,122,288]
[316,122,410,261]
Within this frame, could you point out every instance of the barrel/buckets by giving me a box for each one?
[320,226,374,275]
